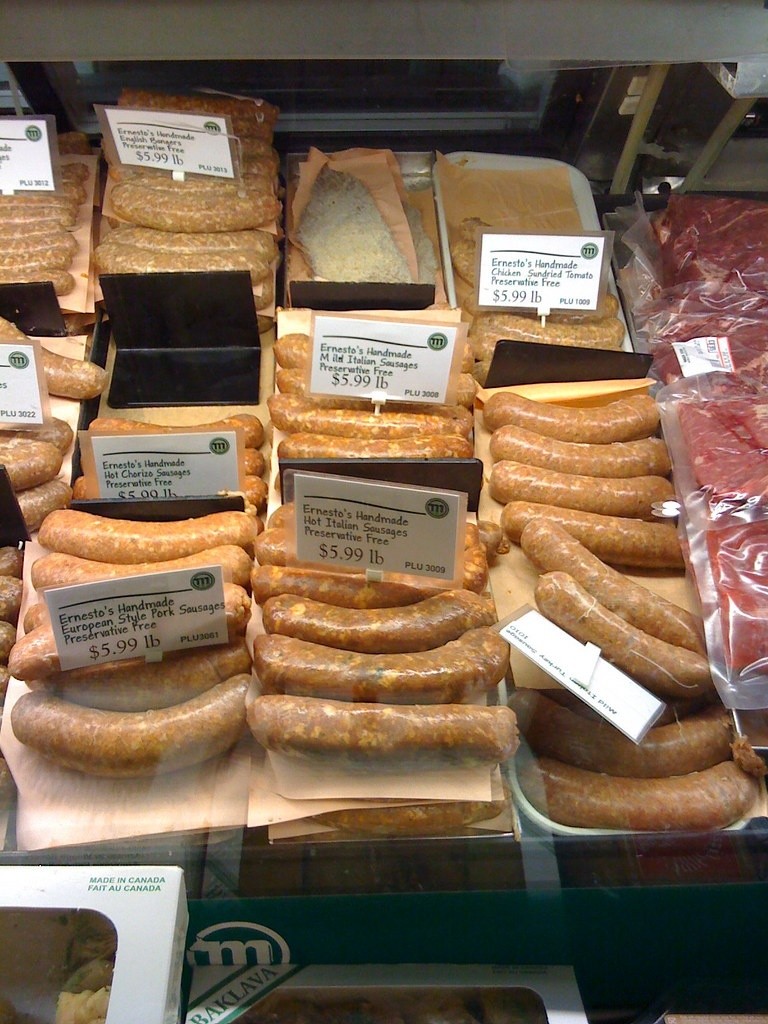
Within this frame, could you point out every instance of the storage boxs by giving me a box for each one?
[0,865,590,1024]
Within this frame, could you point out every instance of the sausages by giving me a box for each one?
[0,96,752,831]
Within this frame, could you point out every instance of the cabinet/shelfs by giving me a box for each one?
[0,0,768,197]
